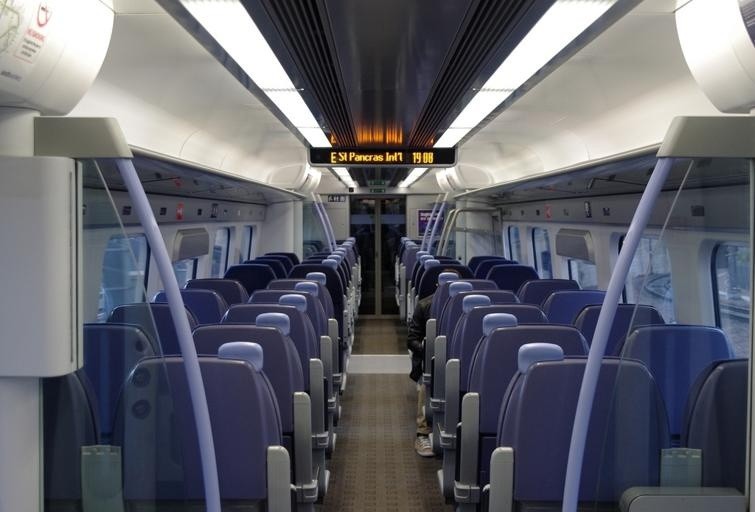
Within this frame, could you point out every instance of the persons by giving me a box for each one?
[406,268,465,460]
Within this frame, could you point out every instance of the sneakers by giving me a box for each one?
[414,436,435,456]
